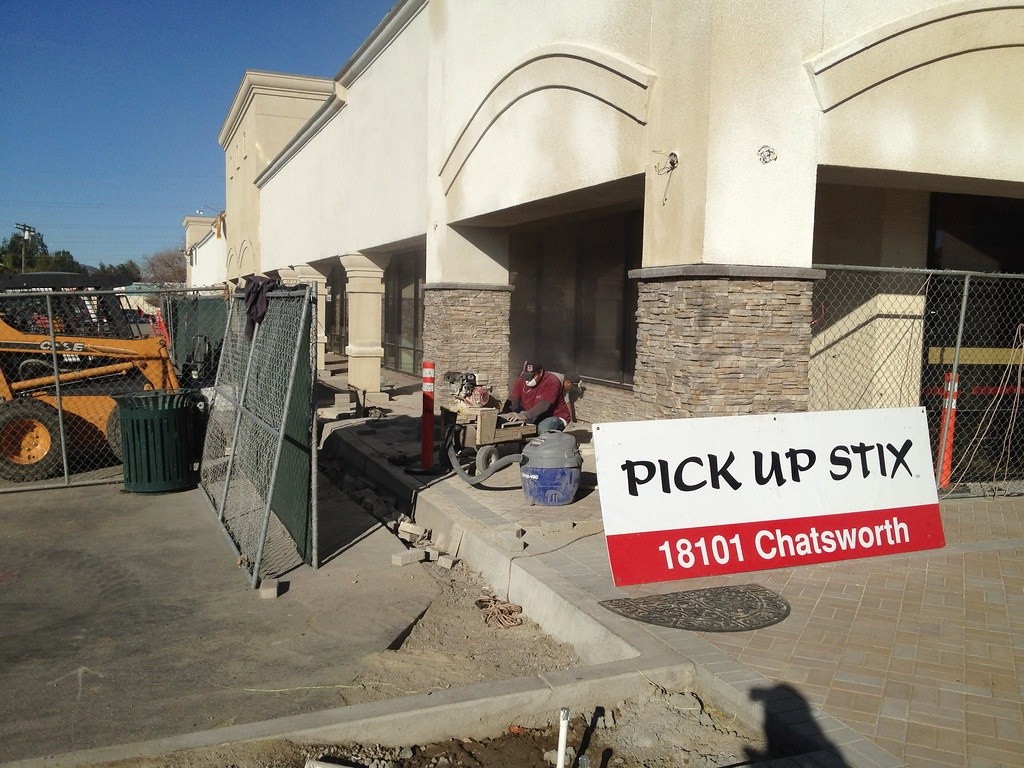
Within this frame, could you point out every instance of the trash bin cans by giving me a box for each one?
[108,388,201,493]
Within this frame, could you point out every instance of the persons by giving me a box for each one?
[495,360,570,436]
[547,371,584,423]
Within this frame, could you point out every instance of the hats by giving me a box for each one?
[520,360,542,381]
[566,371,580,383]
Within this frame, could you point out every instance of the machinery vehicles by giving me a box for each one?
[0,271,185,486]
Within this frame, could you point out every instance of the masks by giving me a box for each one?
[525,375,539,387]
